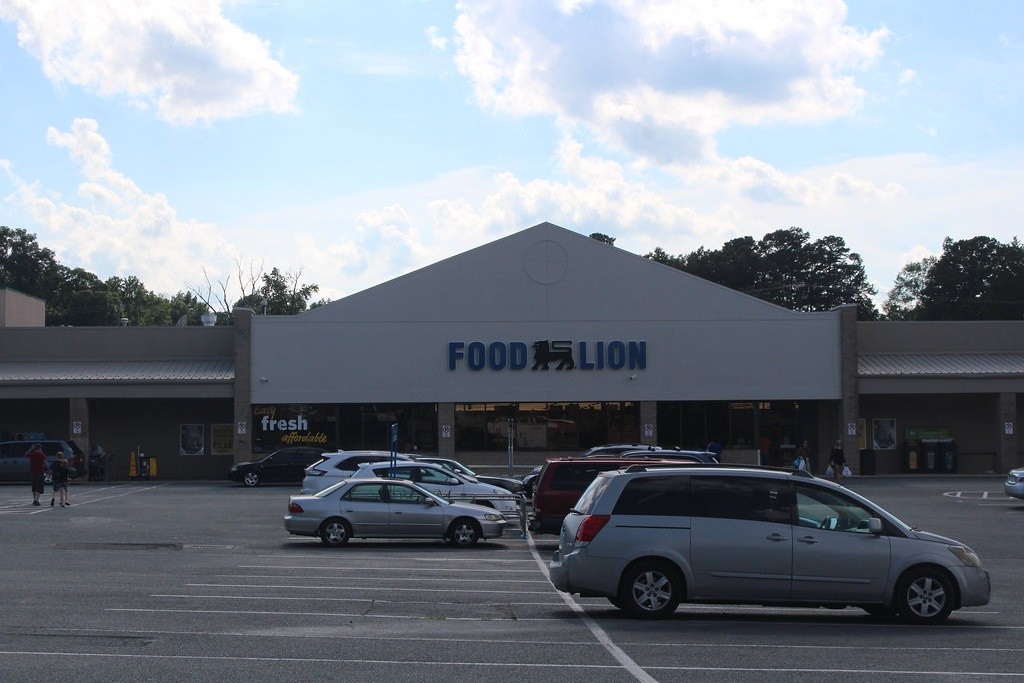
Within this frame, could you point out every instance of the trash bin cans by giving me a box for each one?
[939,438,956,473]
[921,439,940,473]
[904,439,923,473]
[139,456,150,476]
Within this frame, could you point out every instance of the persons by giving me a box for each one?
[828,439,848,485]
[24,442,51,506]
[50,450,67,507]
[60,459,71,506]
[795,439,810,474]
[89,441,107,478]
[708,438,722,464]
[759,431,772,458]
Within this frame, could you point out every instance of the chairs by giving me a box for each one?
[379,486,391,502]
[750,485,769,506]
[410,468,421,482]
[768,485,798,522]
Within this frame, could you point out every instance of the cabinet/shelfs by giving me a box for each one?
[906,442,958,474]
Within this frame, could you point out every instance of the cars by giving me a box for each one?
[284,478,506,544]
[350,461,516,517]
[410,458,523,496]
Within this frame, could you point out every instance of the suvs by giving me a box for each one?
[0,439,85,484]
[529,458,707,533]
[544,465,990,625]
[230,447,329,486]
[301,450,455,495]
[521,444,660,495]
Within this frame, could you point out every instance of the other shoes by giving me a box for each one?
[32,501,37,505]
[34,502,41,505]
[60,501,70,507]
[50,498,55,506]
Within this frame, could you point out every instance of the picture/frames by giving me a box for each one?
[873,419,897,450]
[857,419,867,449]
[211,425,233,455]
[180,423,204,455]
